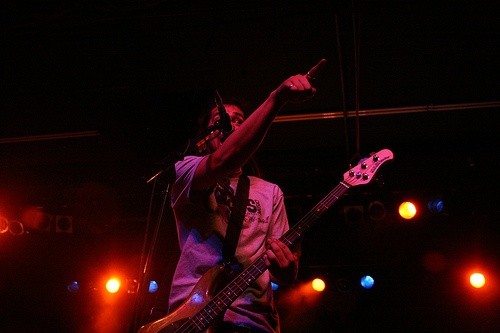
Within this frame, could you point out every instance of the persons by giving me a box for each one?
[169,58,327,333]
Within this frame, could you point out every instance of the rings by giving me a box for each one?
[307,71,315,80]
[287,84,294,90]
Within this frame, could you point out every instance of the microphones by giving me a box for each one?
[214,89,235,137]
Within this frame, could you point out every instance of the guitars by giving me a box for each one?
[136,149,394,333]
